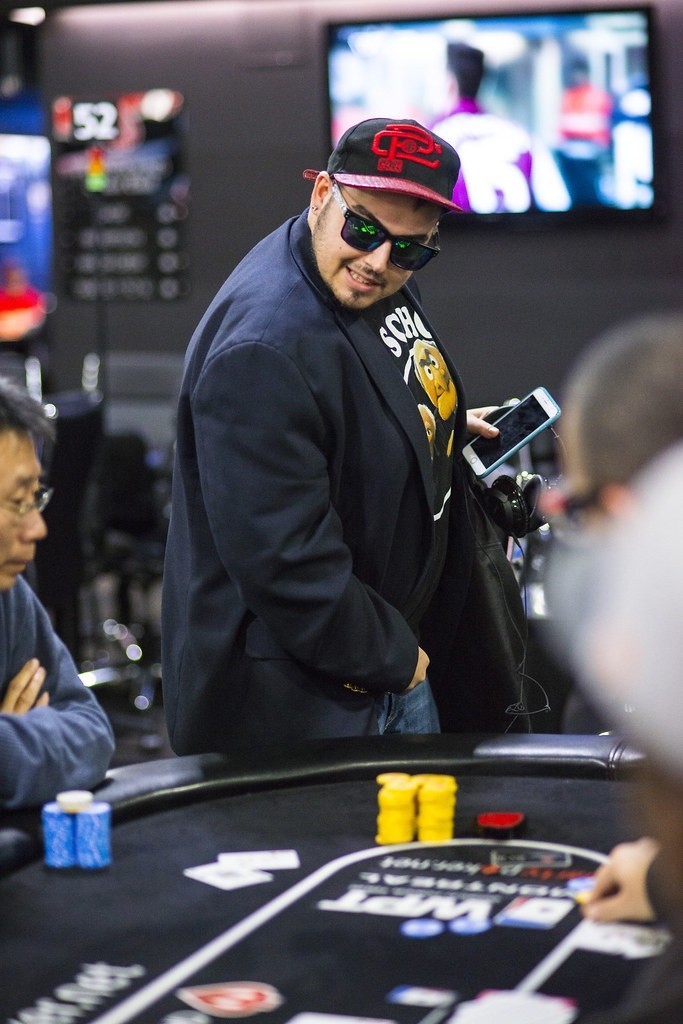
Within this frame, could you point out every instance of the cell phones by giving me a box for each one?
[462,385,561,479]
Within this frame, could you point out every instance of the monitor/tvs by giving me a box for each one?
[319,2,665,228]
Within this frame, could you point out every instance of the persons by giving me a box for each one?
[104,426,165,549]
[163,111,525,760]
[557,58,612,209]
[423,40,573,214]
[543,310,683,934]
[0,382,116,809]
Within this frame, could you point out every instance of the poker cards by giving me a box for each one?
[574,921,668,957]
[510,896,575,927]
[182,849,300,891]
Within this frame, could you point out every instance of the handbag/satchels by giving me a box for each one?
[423,475,538,737]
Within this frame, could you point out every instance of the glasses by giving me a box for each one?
[0,485,54,515]
[332,181,439,271]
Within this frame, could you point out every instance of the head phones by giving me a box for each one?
[462,404,568,538]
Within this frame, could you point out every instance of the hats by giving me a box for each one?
[551,321,683,501]
[326,119,465,213]
